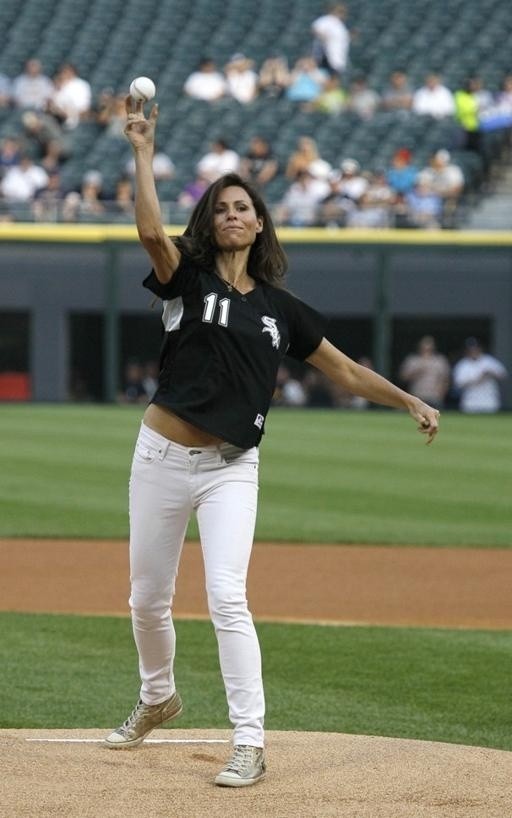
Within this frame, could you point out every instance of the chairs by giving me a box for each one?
[0,1,512,225]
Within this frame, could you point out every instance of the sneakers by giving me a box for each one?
[106,689,183,746]
[214,742,268,790]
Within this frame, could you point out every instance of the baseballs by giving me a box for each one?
[129,76,156,104]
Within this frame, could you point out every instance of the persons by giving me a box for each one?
[105,92,439,786]
